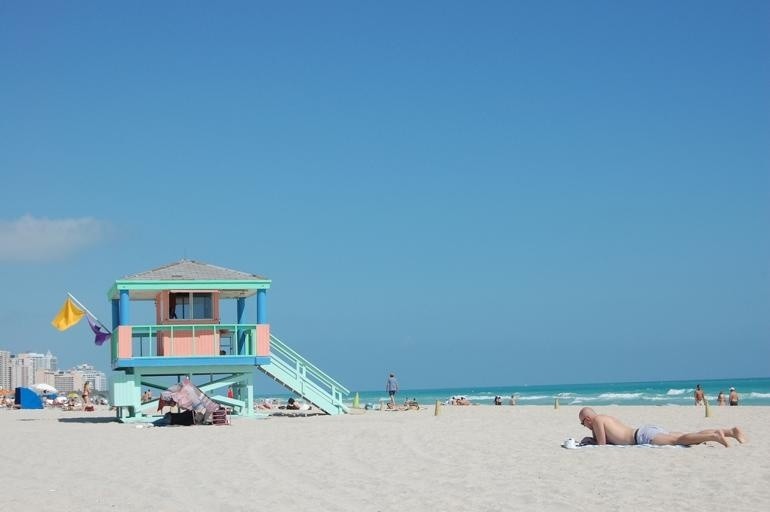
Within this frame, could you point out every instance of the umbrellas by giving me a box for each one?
[31,384,79,398]
[0,389,16,395]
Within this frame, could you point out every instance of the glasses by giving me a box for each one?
[581,418,586,425]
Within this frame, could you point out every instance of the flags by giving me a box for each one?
[50,295,85,332]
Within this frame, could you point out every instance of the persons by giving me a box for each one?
[451,396,469,405]
[386,374,398,405]
[93,395,97,404]
[143,389,152,403]
[220,349,226,355]
[577,406,748,448]
[287,398,302,410]
[494,395,502,405]
[718,391,726,406]
[510,394,516,405]
[2,396,13,407]
[82,381,91,411]
[227,385,233,398]
[169,307,177,319]
[729,387,740,406]
[694,384,706,406]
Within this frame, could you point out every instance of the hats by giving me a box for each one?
[729,387,735,391]
